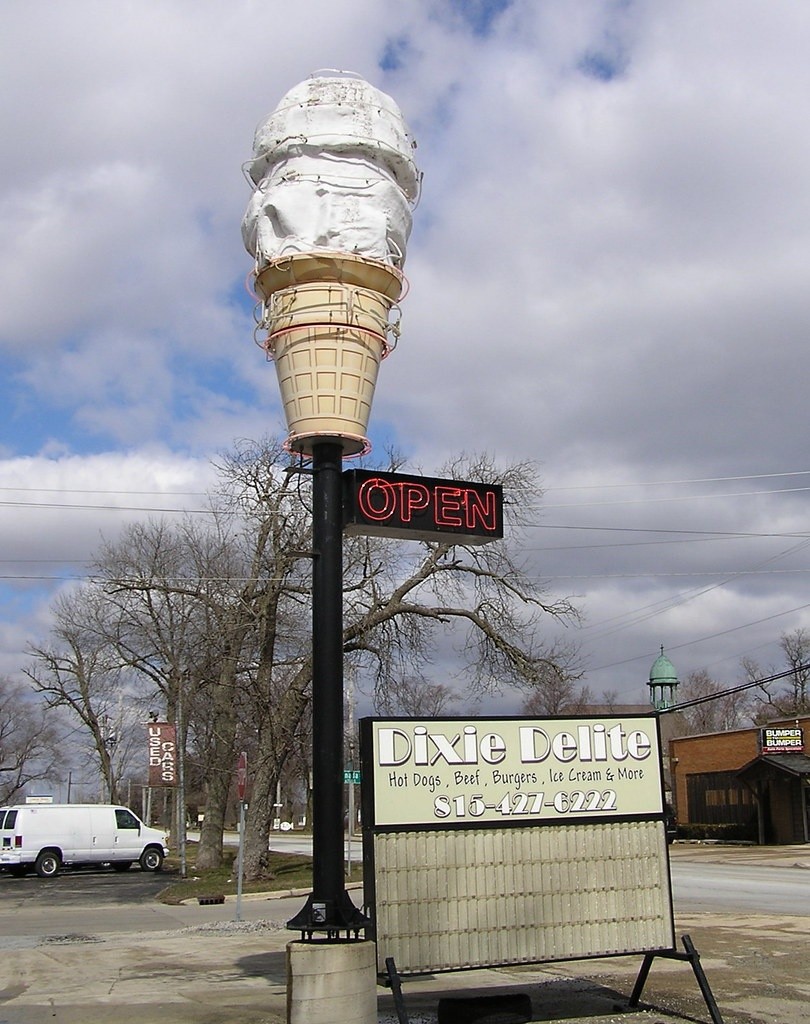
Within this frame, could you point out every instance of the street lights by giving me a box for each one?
[67,781,92,804]
[121,777,130,809]
[160,663,192,879]
[106,736,117,804]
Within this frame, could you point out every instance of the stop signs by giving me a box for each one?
[238,752,248,798]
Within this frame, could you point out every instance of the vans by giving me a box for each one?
[0,804,168,877]
